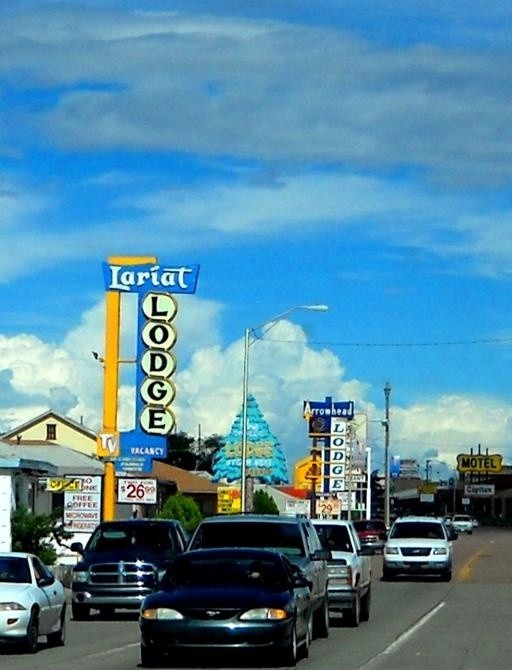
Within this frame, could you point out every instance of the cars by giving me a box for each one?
[138,548,312,667]
[0,552,67,653]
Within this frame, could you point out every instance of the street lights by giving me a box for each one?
[384,381,393,529]
[237,304,328,512]
[347,419,388,520]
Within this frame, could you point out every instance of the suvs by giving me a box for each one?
[307,518,374,627]
[71,518,188,620]
[450,515,473,536]
[188,510,330,638]
[383,514,457,582]
[354,520,386,553]
